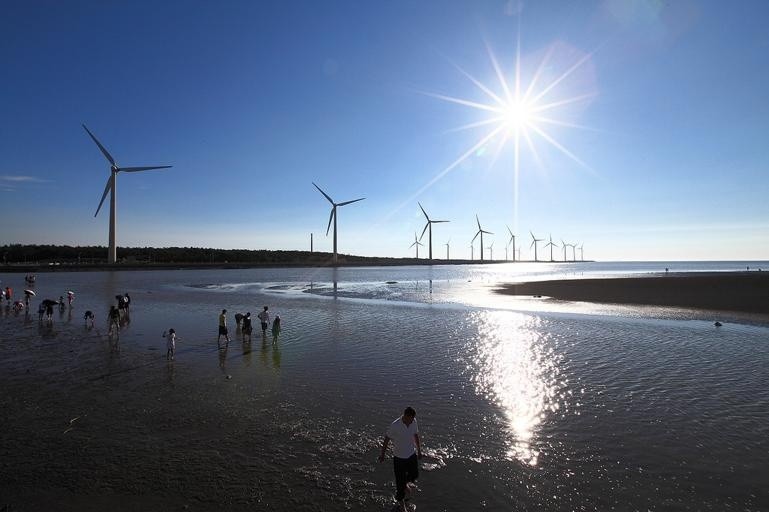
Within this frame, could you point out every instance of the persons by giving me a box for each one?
[218,309,229,345]
[216,333,280,369]
[0,273,132,339]
[258,306,271,332]
[235,313,243,325]
[242,312,253,342]
[378,406,423,512]
[271,315,281,344]
[162,329,176,360]
